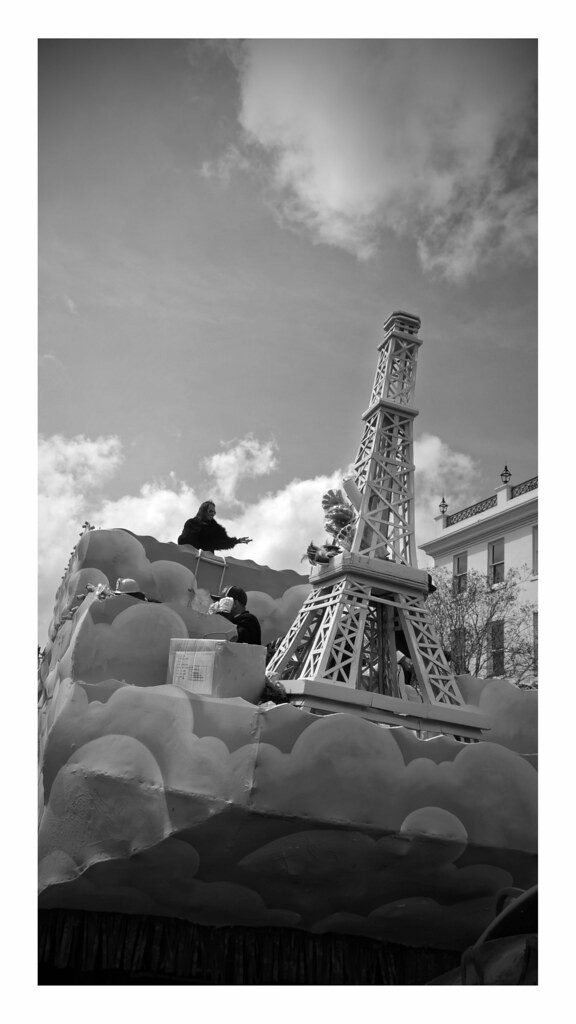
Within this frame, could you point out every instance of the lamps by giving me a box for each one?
[439,498,448,515]
[500,466,512,484]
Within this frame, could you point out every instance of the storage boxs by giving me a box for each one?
[165,638,266,705]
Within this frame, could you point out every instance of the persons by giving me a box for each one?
[178,501,253,554]
[395,574,451,682]
[211,585,261,645]
